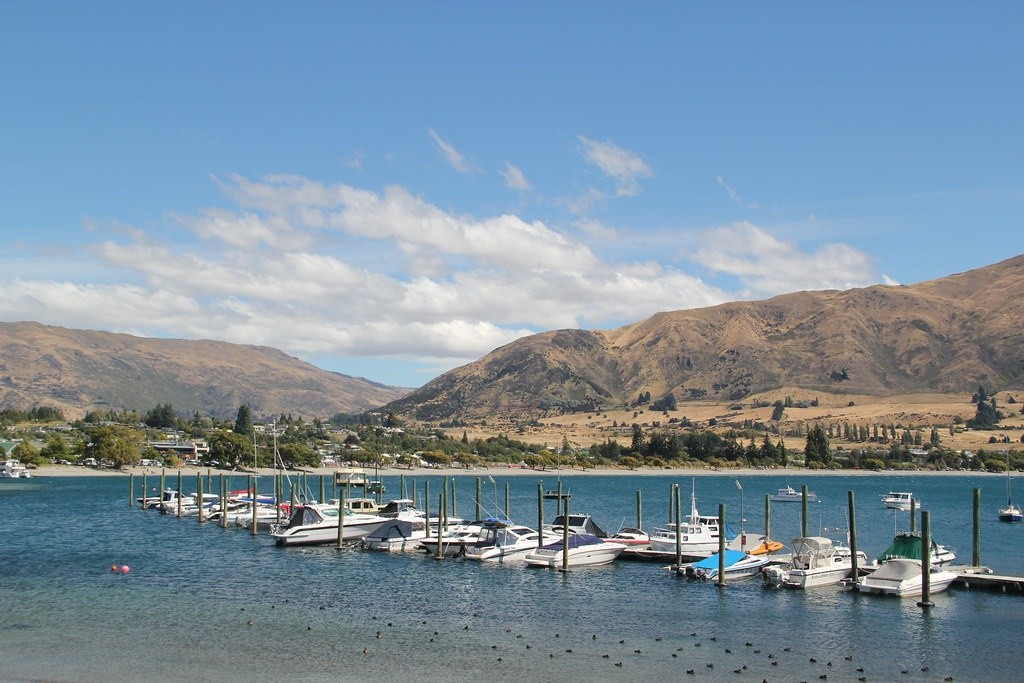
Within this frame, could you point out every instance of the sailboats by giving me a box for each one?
[998,433,1023,522]
[363,451,386,493]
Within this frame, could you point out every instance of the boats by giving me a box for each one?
[648,476,734,551]
[269,502,388,546]
[712,531,784,555]
[770,488,816,502]
[424,516,464,524]
[328,497,388,516]
[880,492,921,511]
[665,549,771,586]
[466,519,563,560]
[525,531,627,568]
[599,528,652,545]
[854,558,958,598]
[362,519,450,554]
[0,460,30,478]
[761,534,852,590]
[418,525,495,558]
[774,537,867,560]
[377,499,426,517]
[136,487,289,524]
[861,530,956,567]
[542,515,608,536]
[333,473,368,485]
